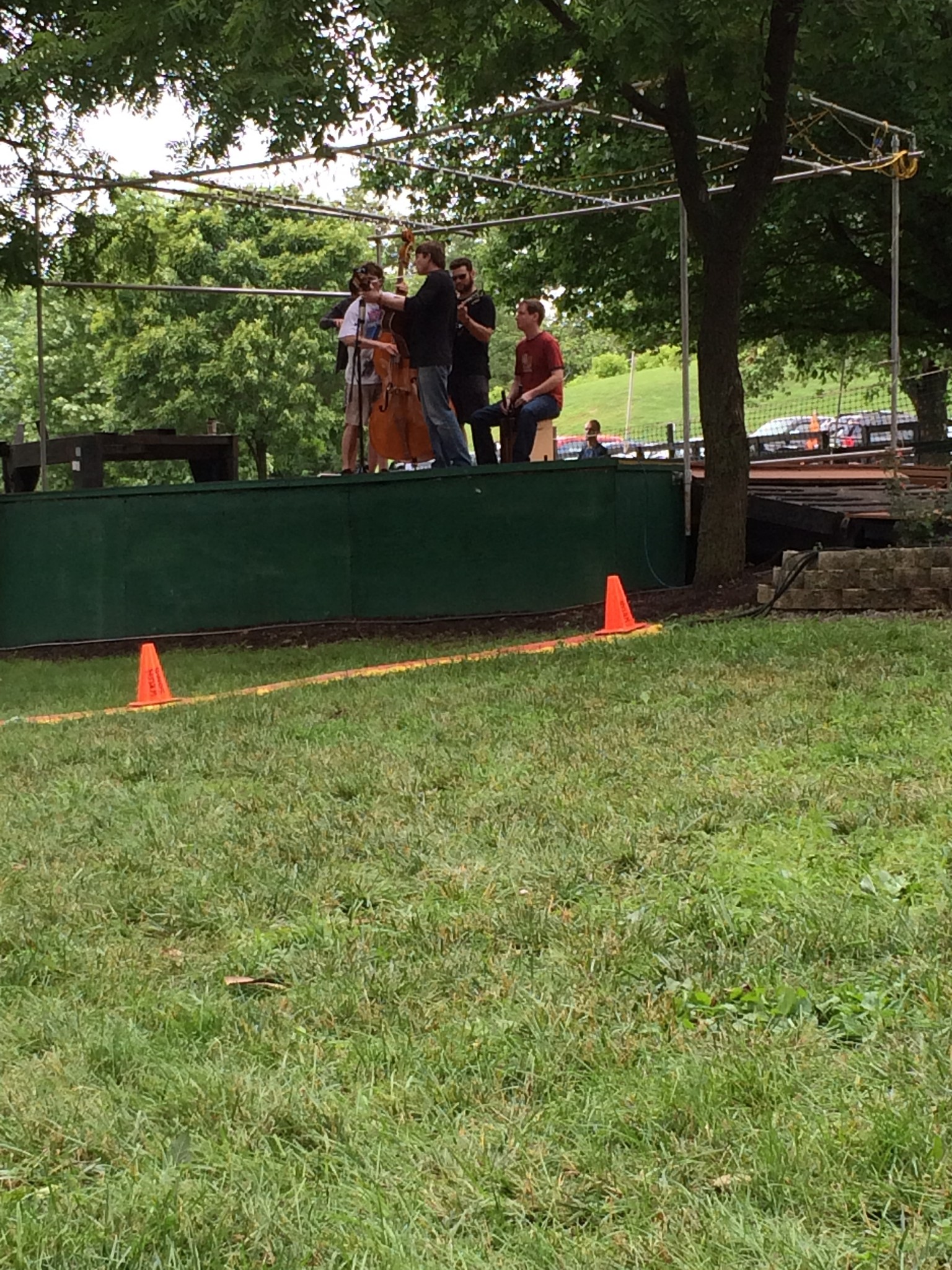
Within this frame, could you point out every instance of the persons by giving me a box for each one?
[319,278,360,372]
[577,419,608,462]
[341,261,409,476]
[449,257,498,464]
[470,298,564,464]
[360,242,472,468]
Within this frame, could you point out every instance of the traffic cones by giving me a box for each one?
[593,575,649,633]
[124,642,185,707]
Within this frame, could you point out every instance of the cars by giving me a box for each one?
[747,414,833,452]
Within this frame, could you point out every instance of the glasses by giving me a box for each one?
[451,273,468,281]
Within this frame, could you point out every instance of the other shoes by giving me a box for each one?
[380,468,389,473]
[342,468,353,474]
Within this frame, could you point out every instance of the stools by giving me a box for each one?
[500,416,554,464]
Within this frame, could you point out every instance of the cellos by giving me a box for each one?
[369,228,455,465]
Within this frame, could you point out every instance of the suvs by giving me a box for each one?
[826,409,952,449]
